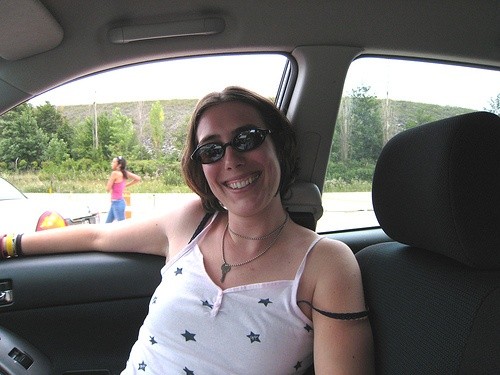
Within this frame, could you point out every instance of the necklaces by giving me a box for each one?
[220,211,289,283]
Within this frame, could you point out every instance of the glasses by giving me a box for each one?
[118,156,122,160]
[190,128,273,164]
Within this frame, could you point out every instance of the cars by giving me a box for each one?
[0,175,69,235]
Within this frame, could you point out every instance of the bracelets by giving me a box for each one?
[16,232,24,257]
[0,234,9,258]
[6,234,15,256]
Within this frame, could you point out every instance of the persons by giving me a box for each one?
[105,155,141,223]
[0,85,377,375]
